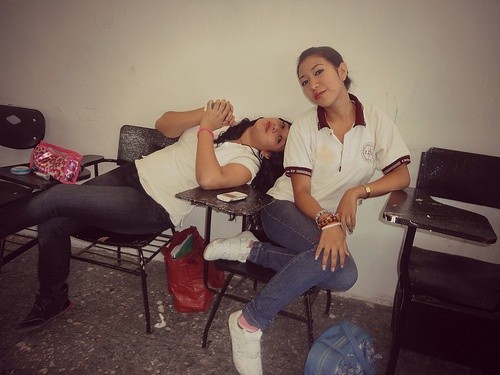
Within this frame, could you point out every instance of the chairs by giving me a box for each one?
[0,104,500,375]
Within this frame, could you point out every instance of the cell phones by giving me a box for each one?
[217,191,248,202]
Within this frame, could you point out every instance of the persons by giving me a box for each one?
[227,46,411,375]
[1,99,292,333]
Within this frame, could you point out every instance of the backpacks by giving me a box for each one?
[304,321,376,375]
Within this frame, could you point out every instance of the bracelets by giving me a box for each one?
[197,129,214,139]
[315,210,341,230]
[362,184,371,199]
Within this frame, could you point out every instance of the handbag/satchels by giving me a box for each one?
[30,140,83,184]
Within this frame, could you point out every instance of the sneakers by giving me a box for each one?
[228,310,263,375]
[203,230,261,263]
[16,293,72,333]
